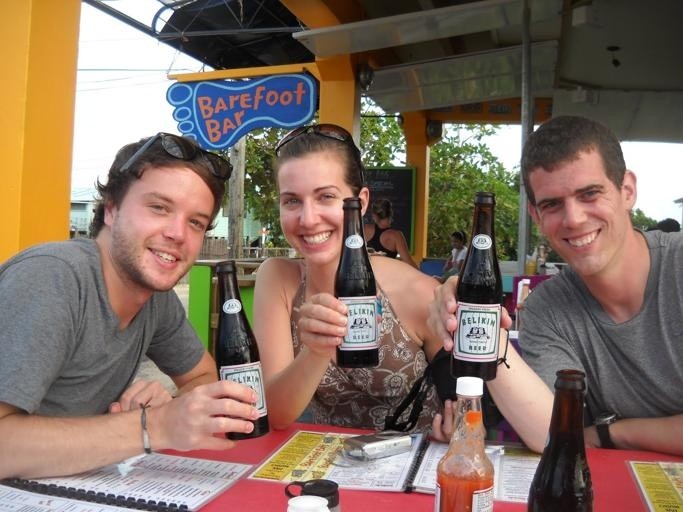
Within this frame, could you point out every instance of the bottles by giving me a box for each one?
[513,278,530,331]
[211,260,271,440]
[527,369,595,511]
[536,245,546,276]
[436,374,494,512]
[449,191,502,383]
[331,196,379,369]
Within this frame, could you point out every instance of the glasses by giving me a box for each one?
[120,132,232,180]
[275,123,364,184]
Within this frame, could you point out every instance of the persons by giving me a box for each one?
[359,198,420,270]
[495,239,518,262]
[250,226,270,259]
[429,230,468,284]
[427,115,683,461]
[0,133,261,485]
[250,126,469,444]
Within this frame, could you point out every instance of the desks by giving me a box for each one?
[418,256,554,316]
[1,415,683,511]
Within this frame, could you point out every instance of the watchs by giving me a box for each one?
[593,410,619,450]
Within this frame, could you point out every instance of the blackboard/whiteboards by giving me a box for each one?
[362,166,416,257]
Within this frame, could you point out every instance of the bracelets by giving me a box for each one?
[497,325,512,370]
[135,402,155,454]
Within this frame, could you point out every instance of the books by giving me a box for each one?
[0,447,254,512]
[625,457,681,510]
[318,437,504,500]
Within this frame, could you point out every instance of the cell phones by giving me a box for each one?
[343,430,412,460]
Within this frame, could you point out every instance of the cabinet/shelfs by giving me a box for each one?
[171,266,258,357]
